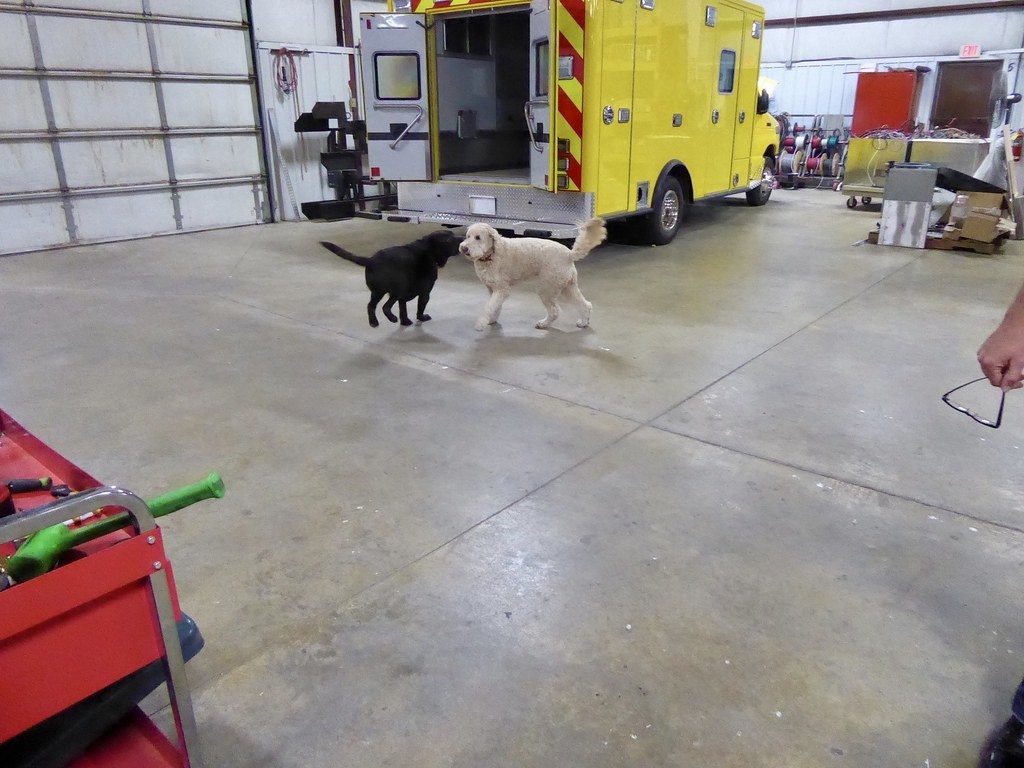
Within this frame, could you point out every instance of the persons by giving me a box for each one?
[978,285,1023,767]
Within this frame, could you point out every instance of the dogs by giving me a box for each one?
[459,218,608,333]
[316,229,466,328]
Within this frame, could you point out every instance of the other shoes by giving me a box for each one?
[988,714,1024,768]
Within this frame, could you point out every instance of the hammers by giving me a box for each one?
[7,472,226,584]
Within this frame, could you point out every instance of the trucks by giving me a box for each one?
[359,0,781,247]
